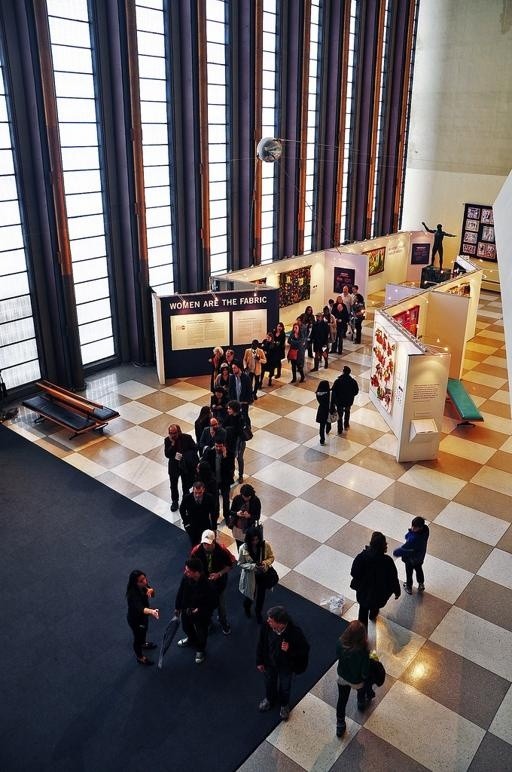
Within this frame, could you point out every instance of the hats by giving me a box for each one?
[200,528,216,545]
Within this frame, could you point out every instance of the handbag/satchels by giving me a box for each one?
[287,349,298,361]
[238,423,254,442]
[326,411,341,424]
[261,566,280,589]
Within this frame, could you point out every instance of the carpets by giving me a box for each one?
[0,420,349,771]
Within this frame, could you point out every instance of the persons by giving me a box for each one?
[123,567,160,666]
[253,604,300,723]
[328,285,365,354]
[164,424,199,512]
[419,219,458,273]
[236,526,277,626]
[194,387,252,524]
[315,380,336,445]
[227,483,262,555]
[350,529,402,636]
[189,526,238,638]
[332,366,359,434]
[393,514,430,597]
[288,306,330,383]
[334,620,372,737]
[209,322,285,412]
[173,556,214,667]
[178,480,218,549]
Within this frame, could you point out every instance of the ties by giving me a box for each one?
[197,498,201,506]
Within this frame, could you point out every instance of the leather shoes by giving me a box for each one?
[241,606,264,622]
[252,373,283,401]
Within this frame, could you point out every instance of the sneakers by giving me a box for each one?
[239,476,244,484]
[279,703,290,721]
[222,623,233,636]
[290,376,306,384]
[170,499,179,512]
[258,697,272,713]
[417,583,425,592]
[194,651,206,664]
[310,353,328,372]
[358,696,372,711]
[336,726,347,738]
[142,643,158,650]
[176,636,192,647]
[402,580,413,594]
[136,655,157,667]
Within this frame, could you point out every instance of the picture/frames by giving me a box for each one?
[410,243,432,265]
[457,202,499,264]
[361,246,387,276]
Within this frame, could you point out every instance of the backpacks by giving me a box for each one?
[292,625,311,675]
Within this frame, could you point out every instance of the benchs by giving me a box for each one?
[41,377,121,437]
[445,377,485,429]
[21,382,103,442]
